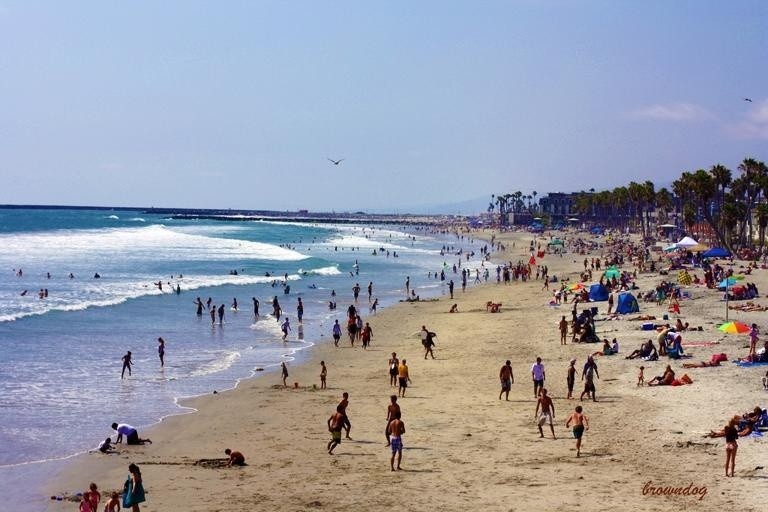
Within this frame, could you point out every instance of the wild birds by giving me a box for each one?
[327,158,345,166]
